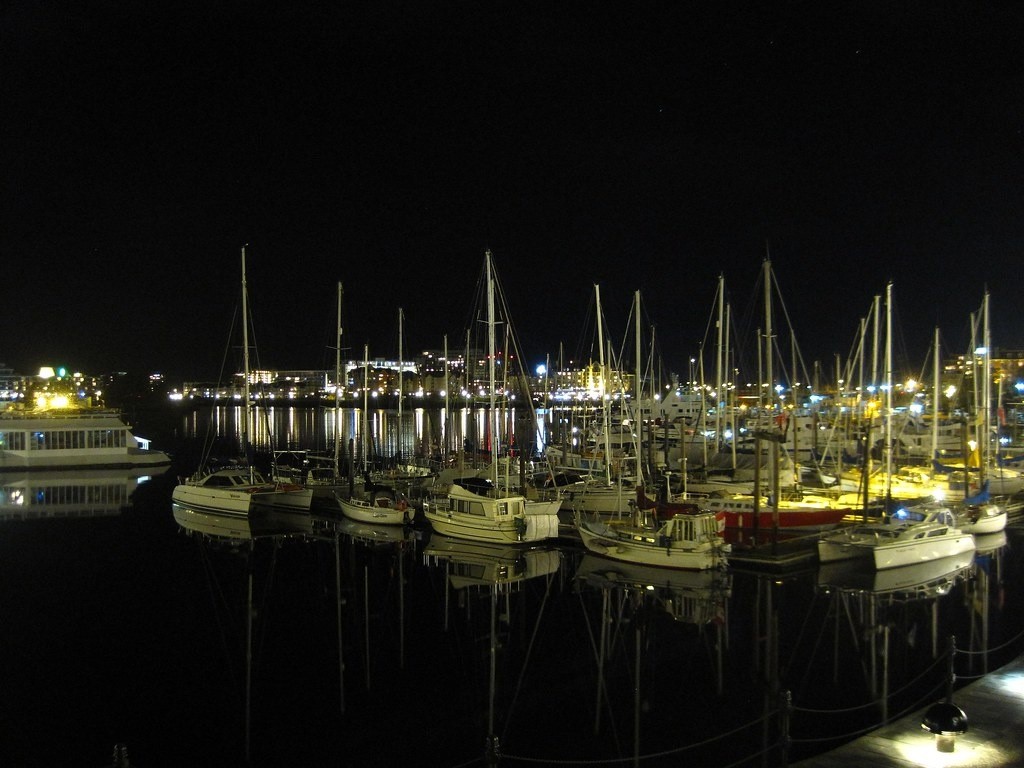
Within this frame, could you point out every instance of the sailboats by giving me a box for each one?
[175,193,1024,571]
[218,534,1024,767]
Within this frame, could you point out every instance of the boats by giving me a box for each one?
[1,362,169,463]
[0,467,168,528]
